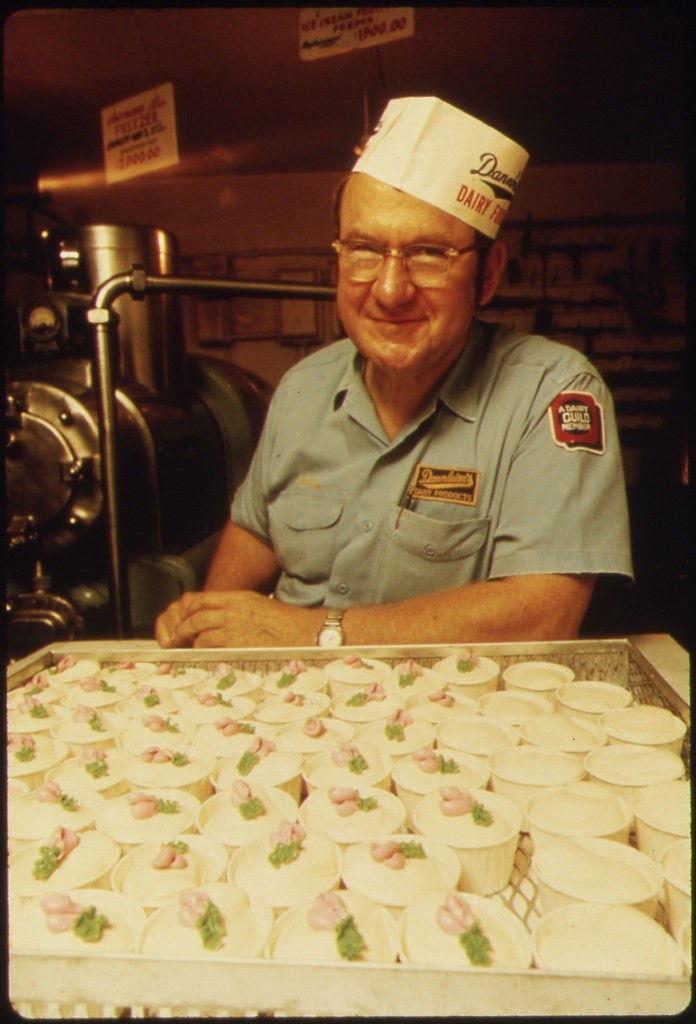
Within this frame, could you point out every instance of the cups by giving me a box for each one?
[8,658,691,976]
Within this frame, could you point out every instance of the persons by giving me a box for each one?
[154,95,636,649]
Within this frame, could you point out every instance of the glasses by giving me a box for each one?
[332,238,485,288]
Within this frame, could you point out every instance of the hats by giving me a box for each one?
[352,95,530,239]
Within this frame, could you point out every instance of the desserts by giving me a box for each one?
[6,648,691,978]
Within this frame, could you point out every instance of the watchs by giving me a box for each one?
[314,608,345,646]
